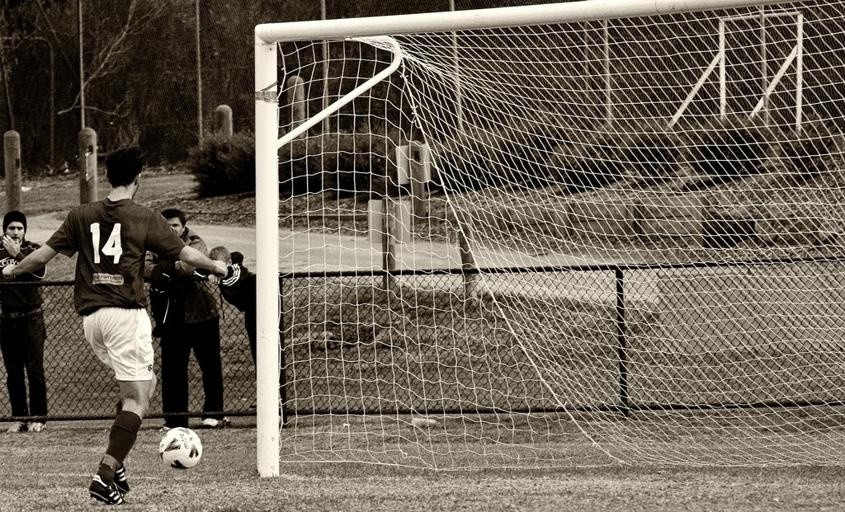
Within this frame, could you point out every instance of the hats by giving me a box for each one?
[3,210,27,233]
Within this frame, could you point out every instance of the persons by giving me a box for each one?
[145,209,223,430]
[2,149,228,504]
[0,211,48,434]
[193,246,291,428]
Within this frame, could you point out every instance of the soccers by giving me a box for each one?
[158,428,204,468]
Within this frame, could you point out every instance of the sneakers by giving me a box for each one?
[89,463,130,505]
[202,418,218,428]
[7,421,46,433]
[158,425,171,433]
